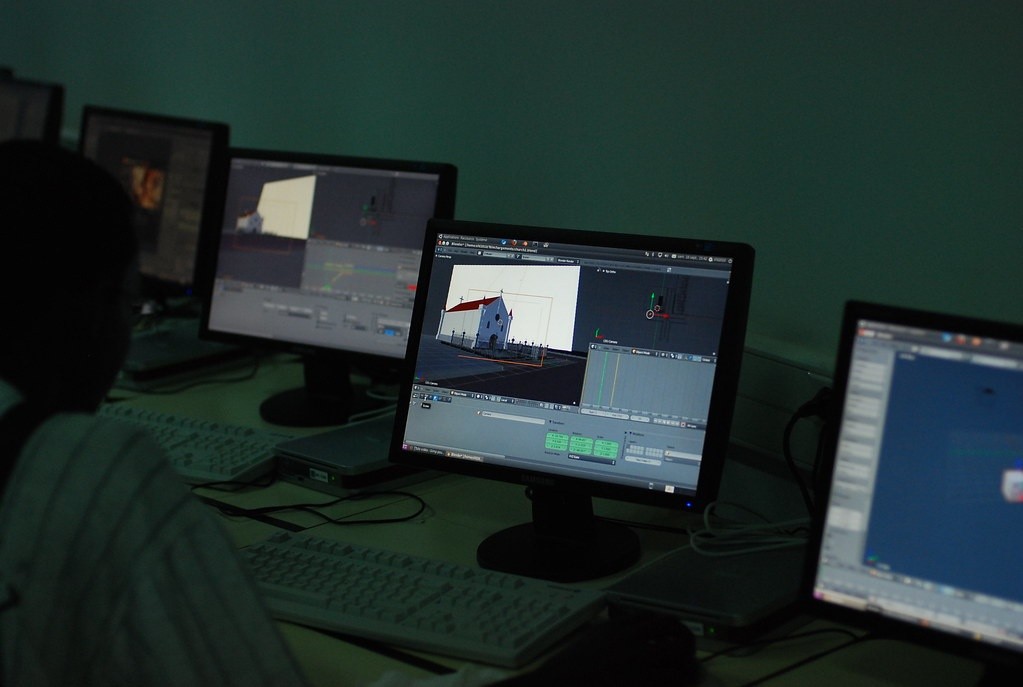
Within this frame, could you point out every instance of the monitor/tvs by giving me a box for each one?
[75,104,230,320]
[0,77,64,147]
[196,147,457,427]
[795,300,1023,686]
[385,219,757,583]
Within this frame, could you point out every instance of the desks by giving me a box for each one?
[105,299,984,687]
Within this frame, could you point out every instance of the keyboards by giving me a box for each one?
[235,529,607,670]
[94,401,297,490]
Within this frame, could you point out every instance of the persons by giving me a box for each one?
[0,138,701,687]
